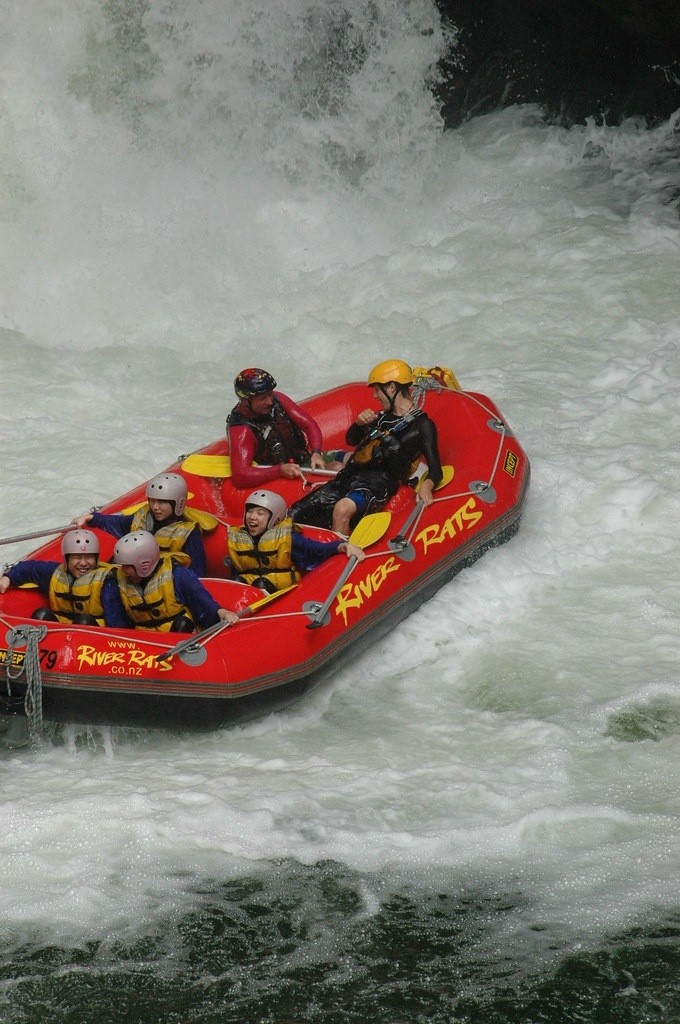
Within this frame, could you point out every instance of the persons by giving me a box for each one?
[69,473,217,577]
[287,359,444,540]
[113,531,239,634]
[226,489,365,594]
[0,529,118,627]
[227,368,354,489]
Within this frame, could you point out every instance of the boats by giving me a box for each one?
[0,361,531,728]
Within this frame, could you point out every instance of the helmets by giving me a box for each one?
[234,368,278,399]
[366,359,413,386]
[243,490,287,531]
[114,530,160,577]
[62,529,100,571]
[146,474,188,516]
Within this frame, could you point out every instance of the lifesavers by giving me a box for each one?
[413,366,461,390]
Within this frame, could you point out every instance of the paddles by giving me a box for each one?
[395,465,454,551]
[0,499,148,545]
[155,582,299,663]
[309,513,393,630]
[182,454,341,478]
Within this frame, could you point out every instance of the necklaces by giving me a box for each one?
[403,402,414,415]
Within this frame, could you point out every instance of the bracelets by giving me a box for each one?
[310,449,323,457]
[337,541,346,553]
